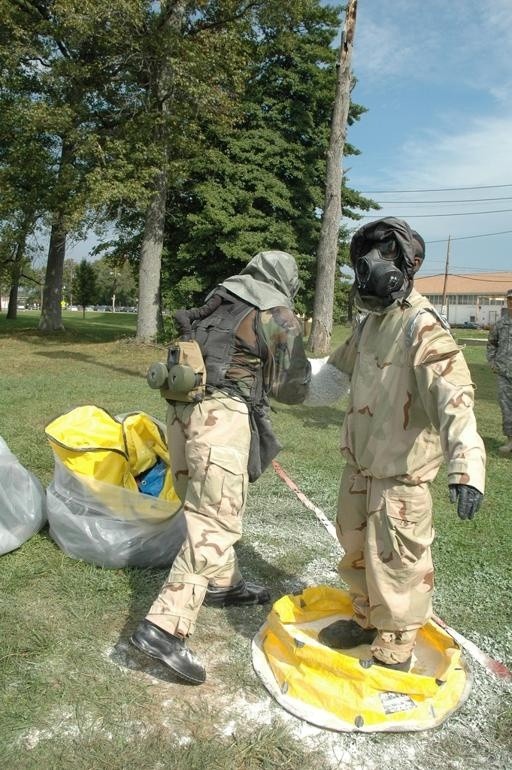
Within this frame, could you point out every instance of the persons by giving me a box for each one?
[302,216,490,673]
[129,249,311,690]
[485,290,510,456]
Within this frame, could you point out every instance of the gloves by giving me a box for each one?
[449,483,484,521]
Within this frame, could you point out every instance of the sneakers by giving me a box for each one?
[203,577,270,608]
[319,618,377,650]
[129,617,207,685]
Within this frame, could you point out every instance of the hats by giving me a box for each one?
[505,289,512,298]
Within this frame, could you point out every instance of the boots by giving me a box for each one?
[497,436,512,456]
